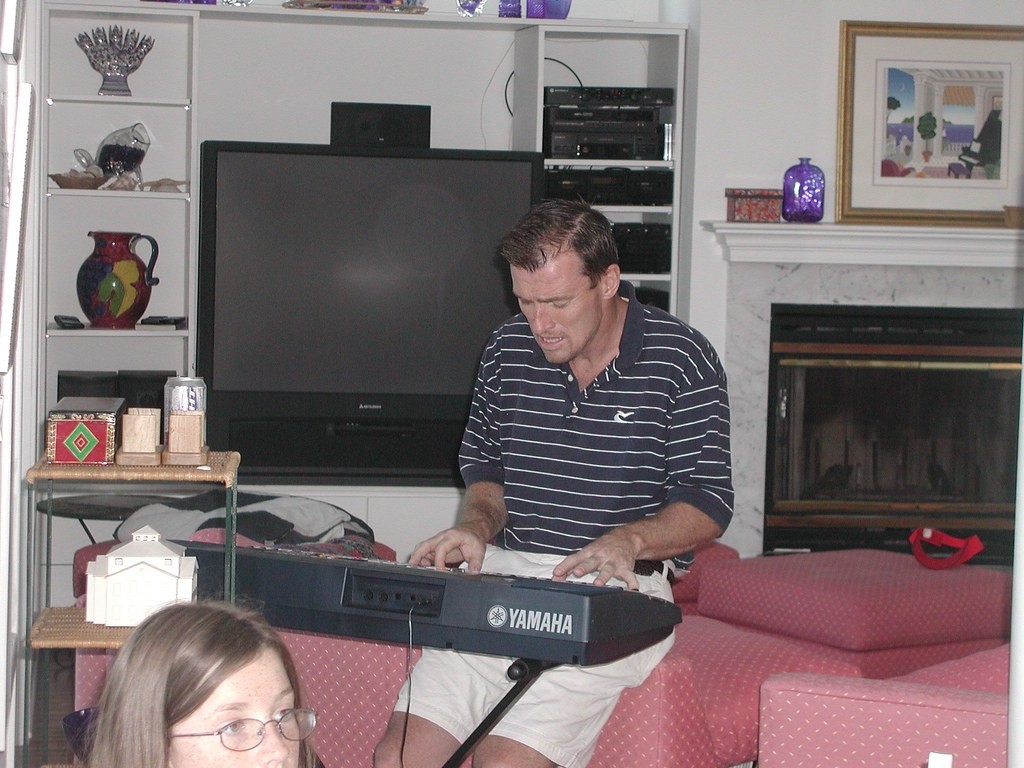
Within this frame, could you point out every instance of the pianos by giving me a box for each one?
[163,539,683,768]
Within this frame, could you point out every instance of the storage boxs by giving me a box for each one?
[724,187,784,222]
[46,396,127,466]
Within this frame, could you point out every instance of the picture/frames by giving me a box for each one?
[834,20,1024,229]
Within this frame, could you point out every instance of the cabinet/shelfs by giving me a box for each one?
[25,449,242,768]
[36,0,689,610]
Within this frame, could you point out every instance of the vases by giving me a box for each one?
[76,231,161,330]
[782,158,826,223]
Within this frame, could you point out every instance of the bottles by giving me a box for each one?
[781,158,825,223]
[77,231,159,330]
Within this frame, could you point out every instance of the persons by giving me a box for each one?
[372,201,734,768]
[87,602,319,768]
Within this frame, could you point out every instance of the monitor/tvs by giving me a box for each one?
[196,141,546,490]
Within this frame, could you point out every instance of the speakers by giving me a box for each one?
[330,101,431,148]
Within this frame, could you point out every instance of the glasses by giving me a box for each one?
[168,709,316,753]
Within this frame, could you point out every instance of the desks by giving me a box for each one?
[35,491,184,544]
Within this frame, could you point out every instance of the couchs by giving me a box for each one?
[68,529,1012,768]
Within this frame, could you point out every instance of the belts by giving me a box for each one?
[638,558,675,586]
